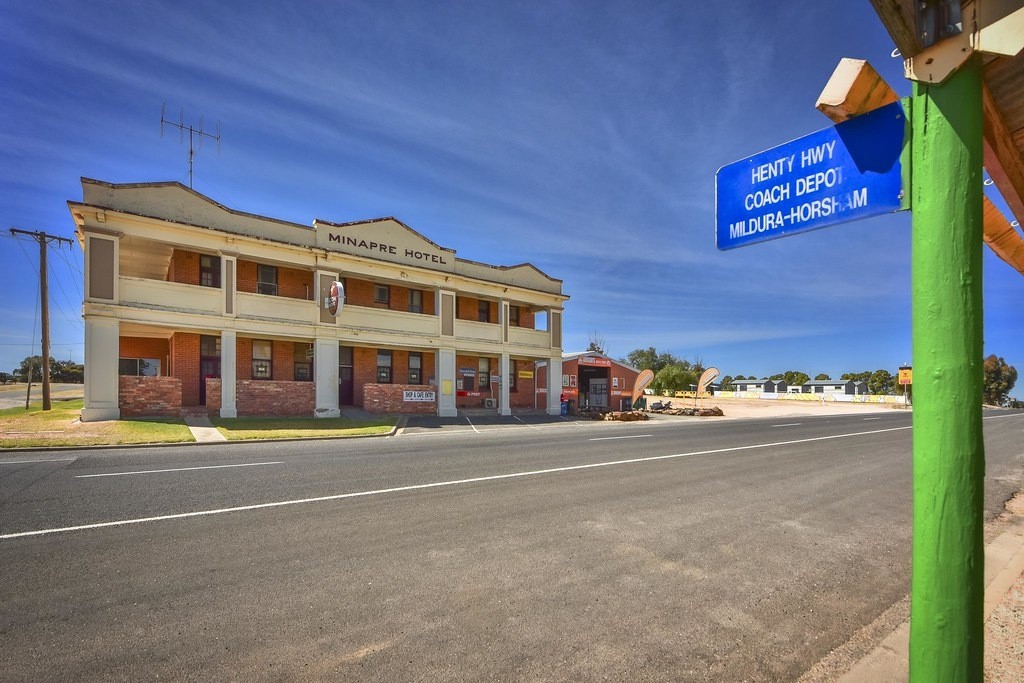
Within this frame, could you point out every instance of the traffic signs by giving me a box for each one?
[712,95,911,251]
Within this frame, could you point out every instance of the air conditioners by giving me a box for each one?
[482,398,497,408]
